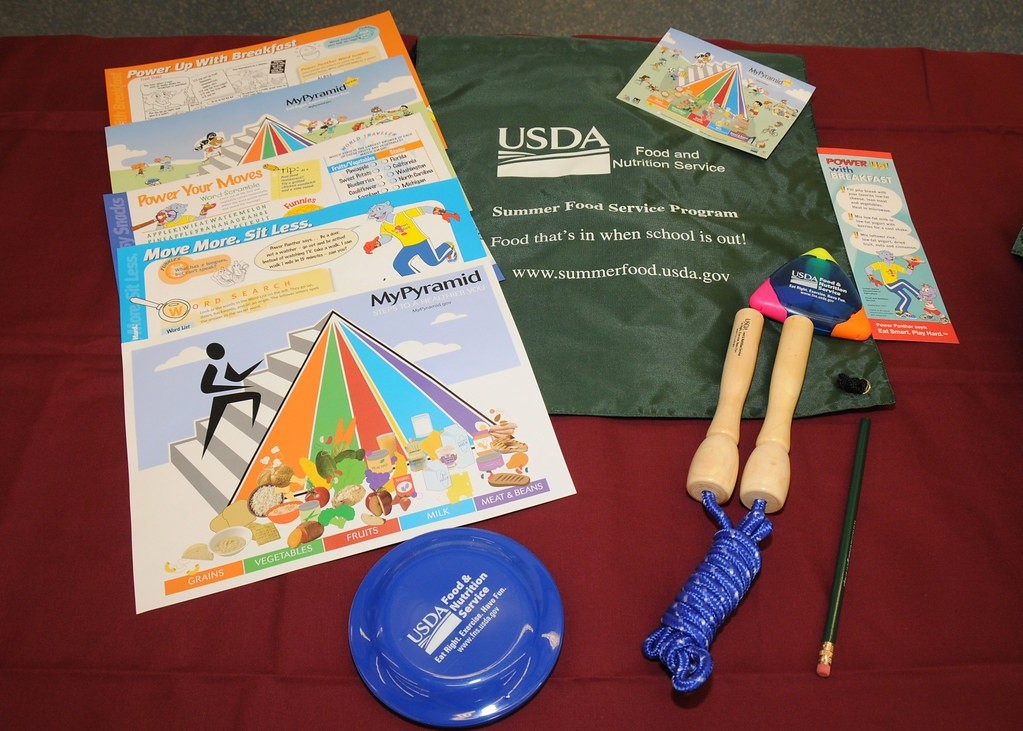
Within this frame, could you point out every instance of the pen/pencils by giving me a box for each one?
[815,417,871,677]
